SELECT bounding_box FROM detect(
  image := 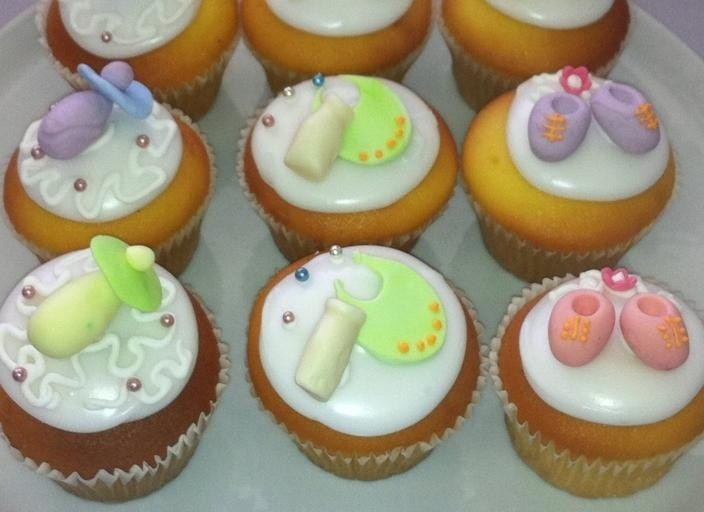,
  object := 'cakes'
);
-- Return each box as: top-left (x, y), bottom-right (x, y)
top-left (236, 71), bottom-right (460, 266)
top-left (1, 234), bottom-right (226, 503)
top-left (240, 0), bottom-right (436, 98)
top-left (438, 1), bottom-right (635, 112)
top-left (3, 62), bottom-right (217, 278)
top-left (461, 65), bottom-right (680, 283)
top-left (242, 244), bottom-right (488, 482)
top-left (34, 1), bottom-right (240, 124)
top-left (489, 267), bottom-right (703, 500)
